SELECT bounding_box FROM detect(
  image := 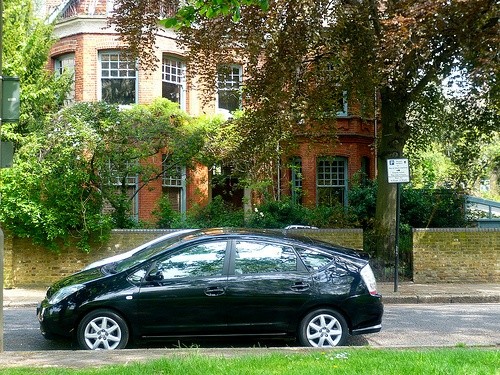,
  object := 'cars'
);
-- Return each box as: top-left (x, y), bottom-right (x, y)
top-left (36, 227), bottom-right (384, 350)
